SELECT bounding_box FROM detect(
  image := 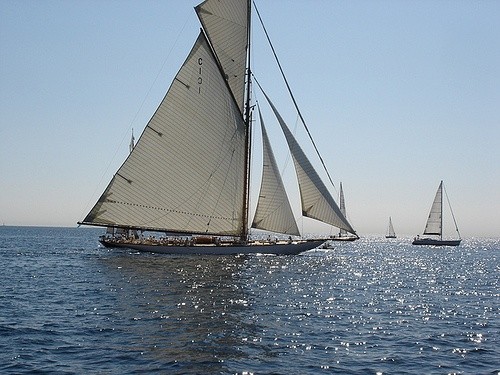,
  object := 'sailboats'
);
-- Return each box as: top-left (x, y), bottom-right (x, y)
top-left (75, 1), bottom-right (360, 257)
top-left (412, 179), bottom-right (463, 246)
top-left (385, 215), bottom-right (397, 238)
top-left (326, 181), bottom-right (360, 241)
